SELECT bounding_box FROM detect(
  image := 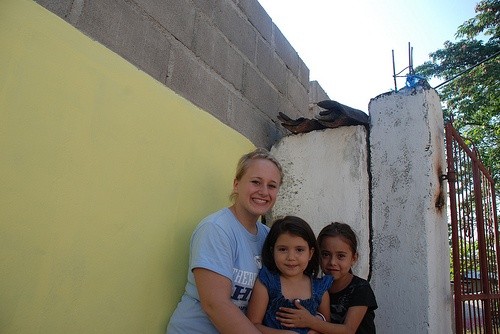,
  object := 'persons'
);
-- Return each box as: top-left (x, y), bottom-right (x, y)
top-left (246, 216), bottom-right (333, 334)
top-left (167, 148), bottom-right (284, 334)
top-left (275, 220), bottom-right (378, 334)
